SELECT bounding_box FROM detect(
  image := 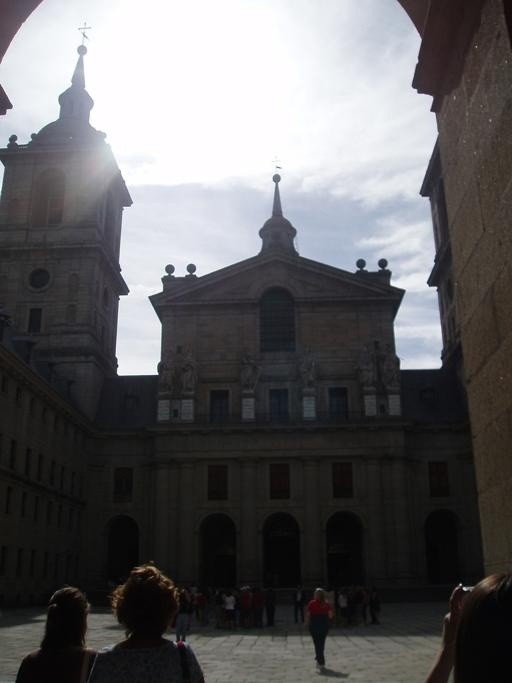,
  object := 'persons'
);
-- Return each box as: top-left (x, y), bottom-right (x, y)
top-left (334, 583), bottom-right (383, 624)
top-left (425, 572), bottom-right (512, 683)
top-left (294, 584), bottom-right (304, 622)
top-left (92, 564), bottom-right (205, 683)
top-left (15, 586), bottom-right (99, 683)
top-left (358, 344), bottom-right (400, 393)
top-left (174, 584), bottom-right (276, 642)
top-left (242, 348), bottom-right (257, 391)
top-left (158, 353), bottom-right (199, 393)
top-left (299, 344), bottom-right (316, 387)
top-left (303, 587), bottom-right (334, 672)
